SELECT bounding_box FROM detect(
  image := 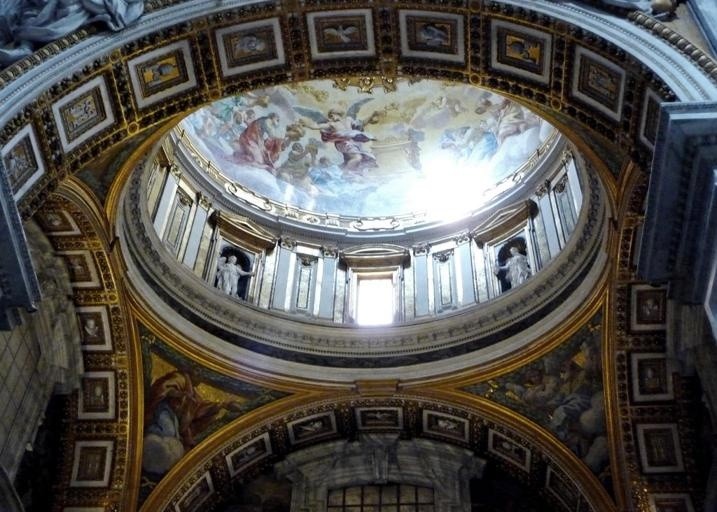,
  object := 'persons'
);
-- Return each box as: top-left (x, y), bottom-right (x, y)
top-left (216, 256), bottom-right (254, 297)
top-left (498, 246), bottom-right (532, 288)
